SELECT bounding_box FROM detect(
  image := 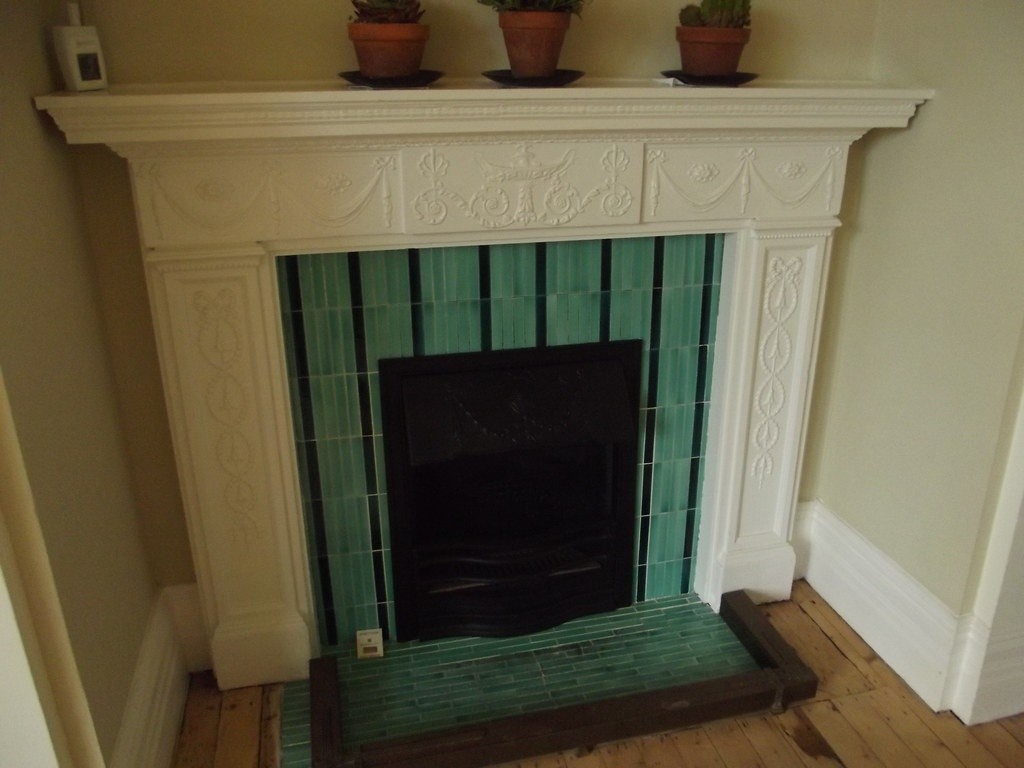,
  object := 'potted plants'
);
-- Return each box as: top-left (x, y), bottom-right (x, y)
top-left (478, 0), bottom-right (583, 76)
top-left (348, 0), bottom-right (431, 77)
top-left (675, 0), bottom-right (751, 77)
